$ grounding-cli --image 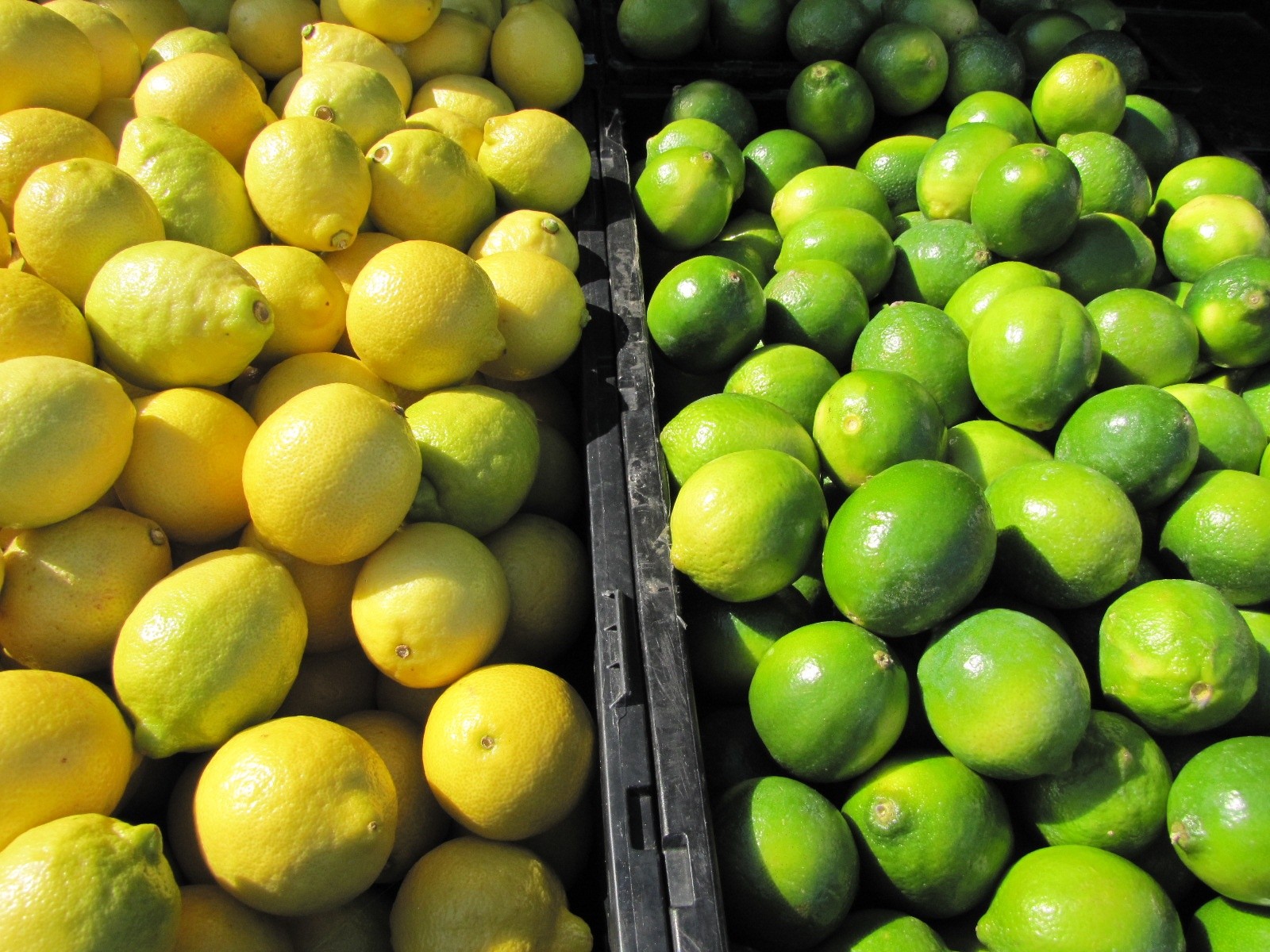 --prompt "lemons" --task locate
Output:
[615,0,1270,952]
[0,0,606,952]
